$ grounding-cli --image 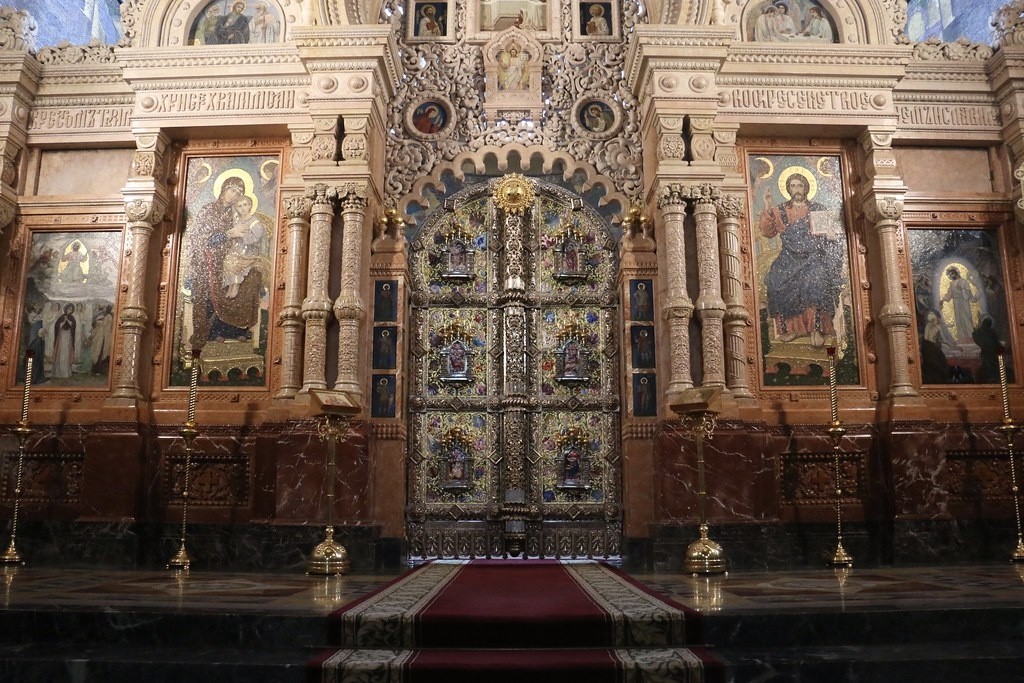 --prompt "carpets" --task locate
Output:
[301,556,726,683]
